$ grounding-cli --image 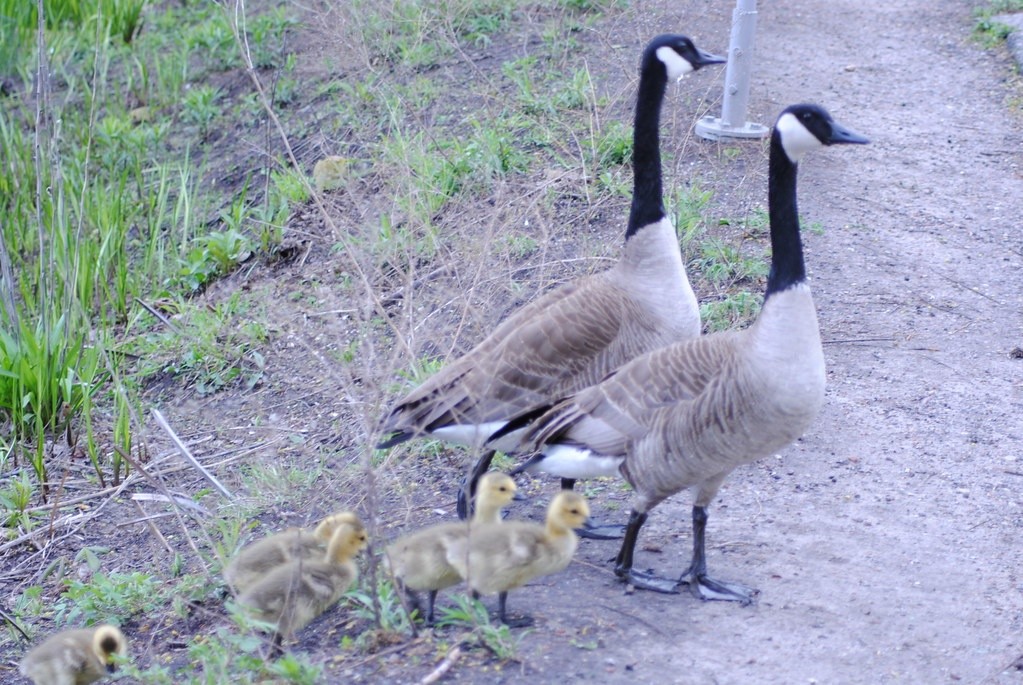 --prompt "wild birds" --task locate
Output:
[217,32,870,645]
[21,623,128,685]
